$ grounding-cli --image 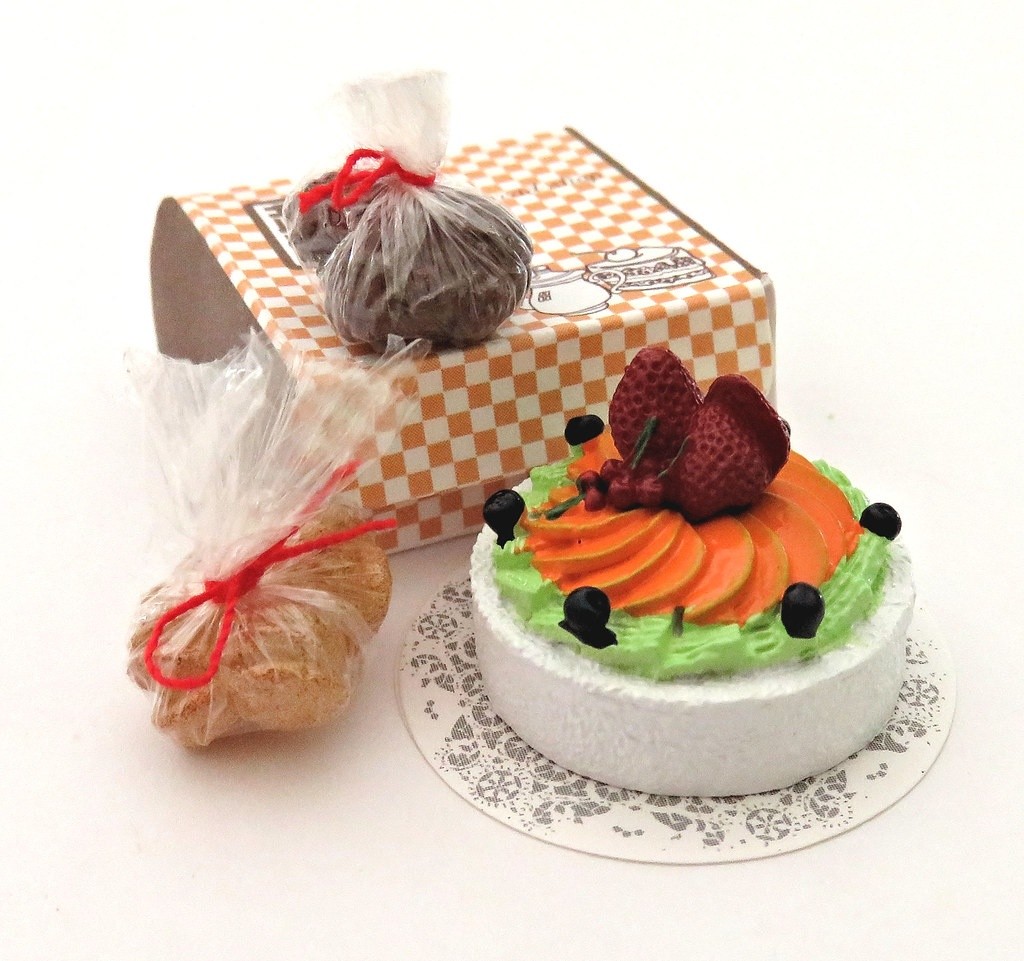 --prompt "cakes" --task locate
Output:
[470,346,915,795]
